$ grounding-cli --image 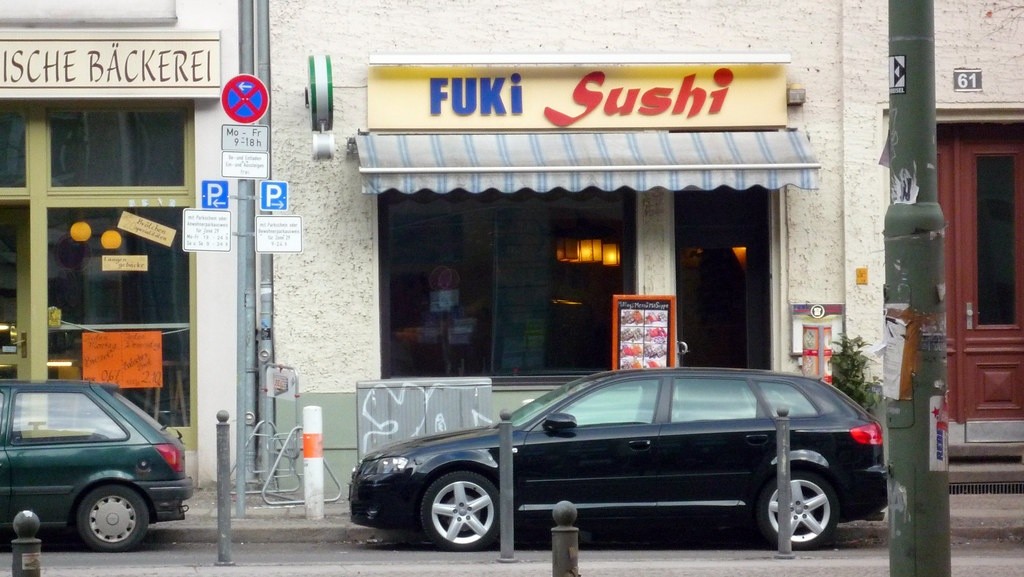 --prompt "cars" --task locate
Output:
[0,375,195,552]
[343,362,892,553]
